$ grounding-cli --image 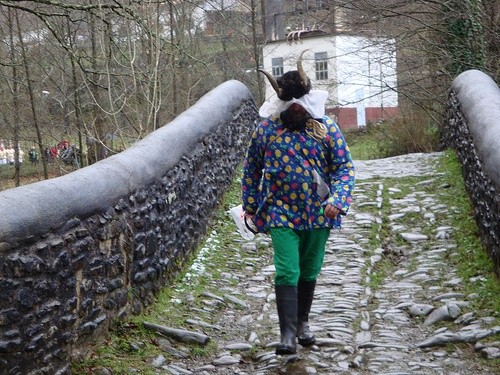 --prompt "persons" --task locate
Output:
[0,137,69,164]
[242,49,355,354]
